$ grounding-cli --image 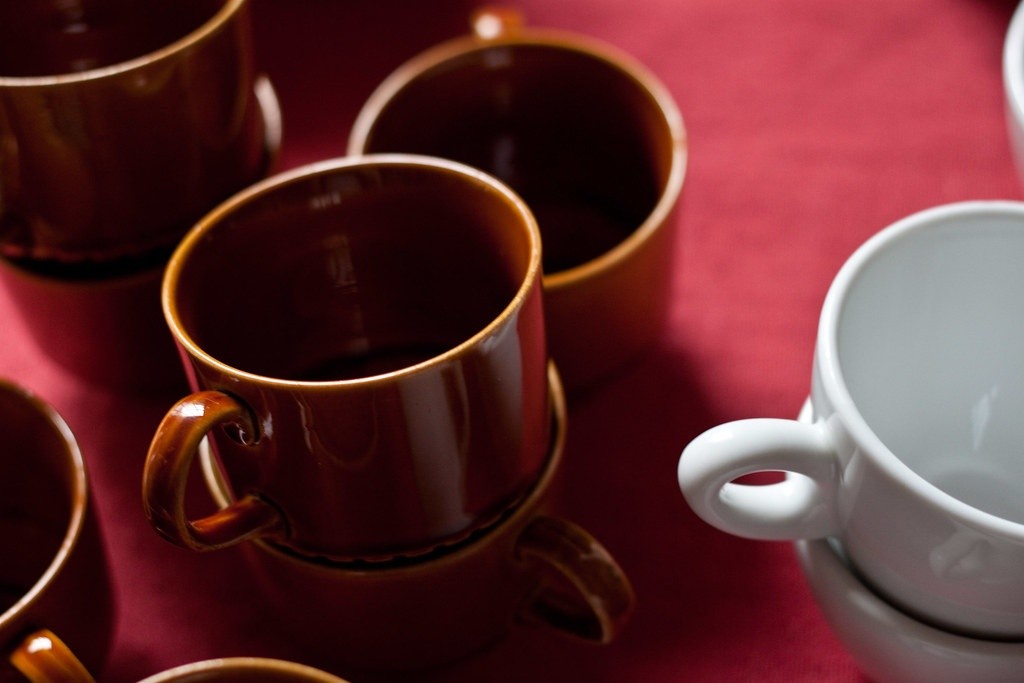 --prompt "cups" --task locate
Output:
[186,362,635,670]
[0,372,125,683]
[141,153,554,571]
[772,385,1024,683]
[0,78,325,395]
[342,10,693,392]
[0,0,260,268]
[136,657,348,683]
[676,198,1024,642]
